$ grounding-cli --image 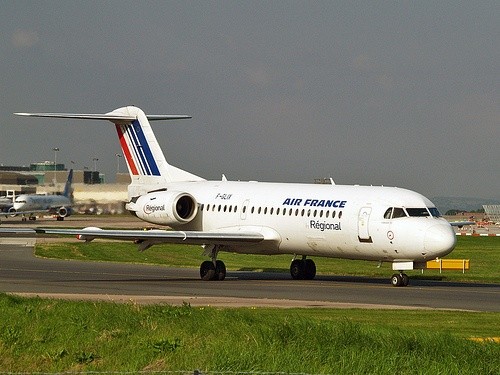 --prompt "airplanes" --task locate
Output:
[0,167,75,219]
[0,104,476,287]
